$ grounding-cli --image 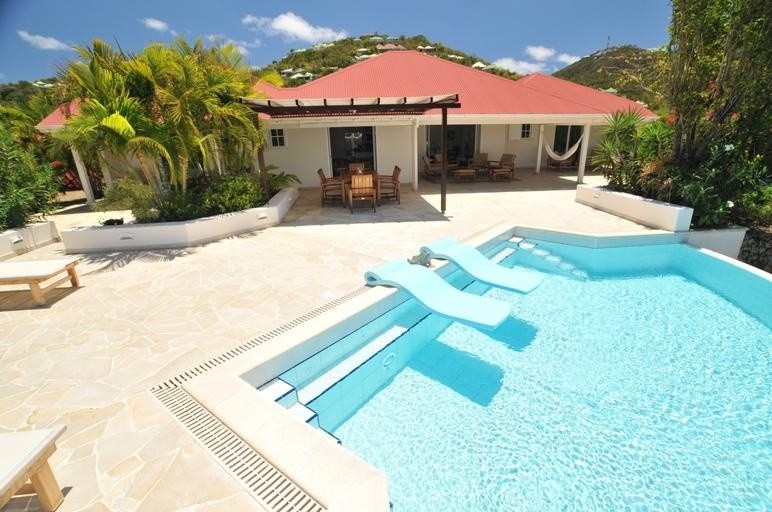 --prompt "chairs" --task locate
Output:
[423,152,517,183]
[0,257,84,306]
[547,152,572,171]
[317,163,402,214]
[0,425,68,512]
